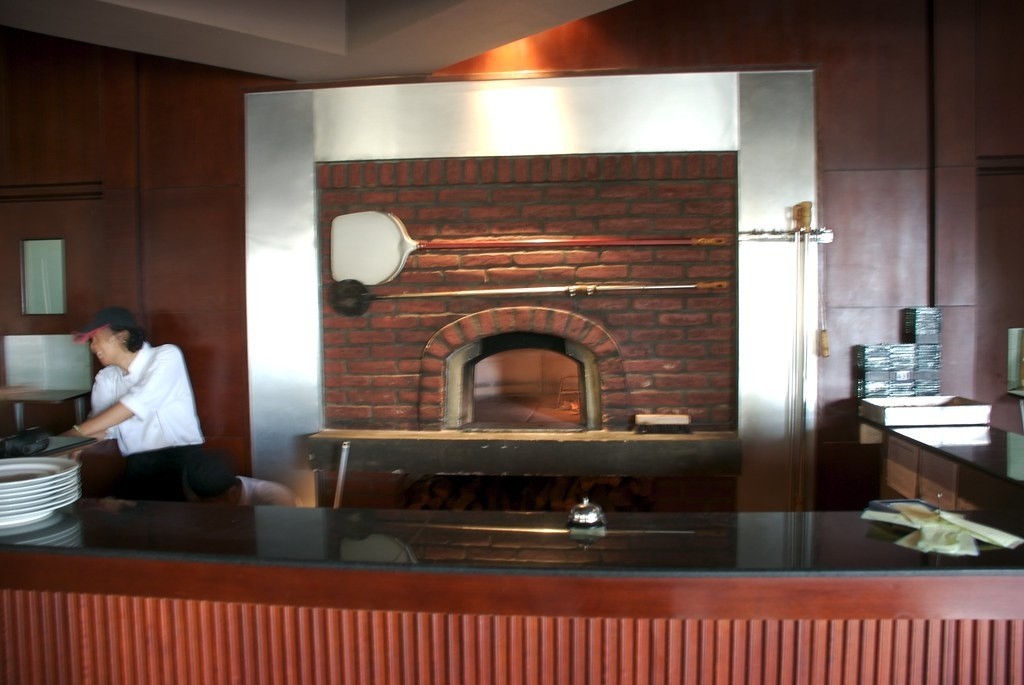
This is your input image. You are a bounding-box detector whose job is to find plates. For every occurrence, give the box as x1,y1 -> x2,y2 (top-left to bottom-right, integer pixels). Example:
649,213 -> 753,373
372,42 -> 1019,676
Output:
0,456 -> 83,528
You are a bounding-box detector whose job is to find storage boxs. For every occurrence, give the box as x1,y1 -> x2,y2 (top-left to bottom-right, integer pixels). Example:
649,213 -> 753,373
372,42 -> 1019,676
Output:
854,307 -> 992,428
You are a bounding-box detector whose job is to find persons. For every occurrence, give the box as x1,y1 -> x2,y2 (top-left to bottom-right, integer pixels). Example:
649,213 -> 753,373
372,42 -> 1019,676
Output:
182,447 -> 302,508
57,307 -> 205,500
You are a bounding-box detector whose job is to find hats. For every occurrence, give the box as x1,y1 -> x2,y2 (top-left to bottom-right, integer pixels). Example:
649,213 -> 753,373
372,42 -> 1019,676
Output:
73,306 -> 140,346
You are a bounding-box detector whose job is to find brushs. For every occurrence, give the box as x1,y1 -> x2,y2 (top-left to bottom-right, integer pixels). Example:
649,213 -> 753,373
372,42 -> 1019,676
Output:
634,414 -> 692,434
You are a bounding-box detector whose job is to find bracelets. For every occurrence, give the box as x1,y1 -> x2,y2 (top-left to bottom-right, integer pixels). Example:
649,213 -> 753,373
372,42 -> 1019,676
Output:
74,425 -> 84,438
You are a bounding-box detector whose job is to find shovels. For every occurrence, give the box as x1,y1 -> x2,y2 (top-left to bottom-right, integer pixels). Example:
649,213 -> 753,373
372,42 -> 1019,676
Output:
329,210 -> 728,286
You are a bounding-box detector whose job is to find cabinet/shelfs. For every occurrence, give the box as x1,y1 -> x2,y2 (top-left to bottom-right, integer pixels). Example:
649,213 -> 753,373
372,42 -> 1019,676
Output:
859,415 -> 1023,513
